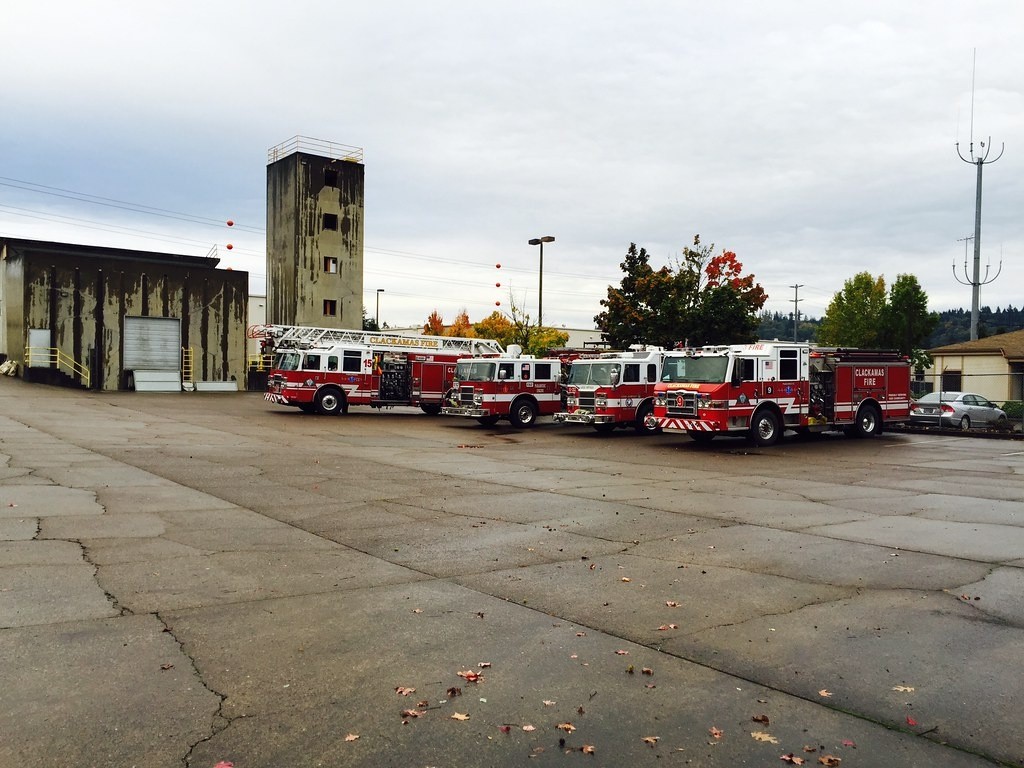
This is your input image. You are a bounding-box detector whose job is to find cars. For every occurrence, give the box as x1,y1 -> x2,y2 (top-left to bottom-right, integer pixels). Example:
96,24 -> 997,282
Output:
909,391 -> 1008,430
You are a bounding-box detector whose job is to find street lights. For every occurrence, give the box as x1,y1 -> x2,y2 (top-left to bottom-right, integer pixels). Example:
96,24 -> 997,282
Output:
528,236 -> 556,325
376,288 -> 385,328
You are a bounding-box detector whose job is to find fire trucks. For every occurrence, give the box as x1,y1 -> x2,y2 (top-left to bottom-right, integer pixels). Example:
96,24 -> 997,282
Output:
553,344 -> 666,436
652,338 -> 913,447
436,344 -> 576,428
246,322 -> 506,416
